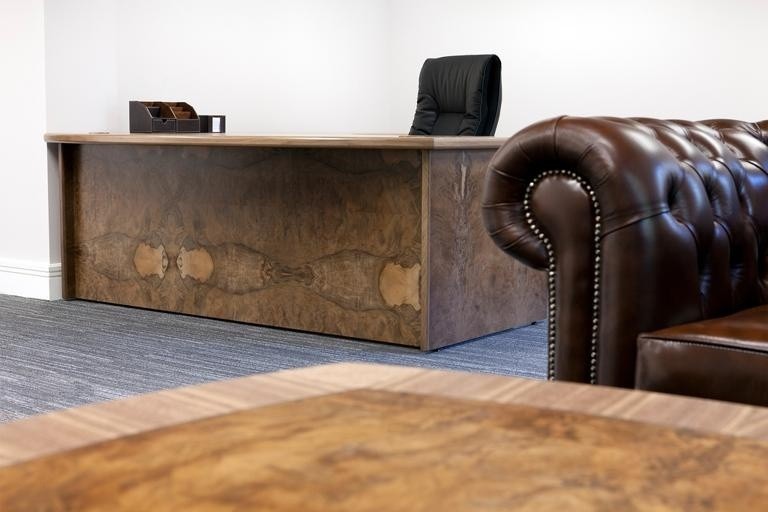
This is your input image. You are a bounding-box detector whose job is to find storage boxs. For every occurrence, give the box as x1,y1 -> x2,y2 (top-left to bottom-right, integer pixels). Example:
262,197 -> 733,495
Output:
129,101 -> 200,133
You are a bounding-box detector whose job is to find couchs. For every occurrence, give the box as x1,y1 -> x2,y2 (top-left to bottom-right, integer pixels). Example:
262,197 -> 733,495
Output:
480,115 -> 768,406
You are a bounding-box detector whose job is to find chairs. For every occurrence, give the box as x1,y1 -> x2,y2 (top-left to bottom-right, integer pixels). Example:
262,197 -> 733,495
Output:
409,54 -> 501,135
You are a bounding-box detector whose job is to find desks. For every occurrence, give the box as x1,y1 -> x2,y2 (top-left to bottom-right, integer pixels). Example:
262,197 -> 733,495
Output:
0,358 -> 768,512
